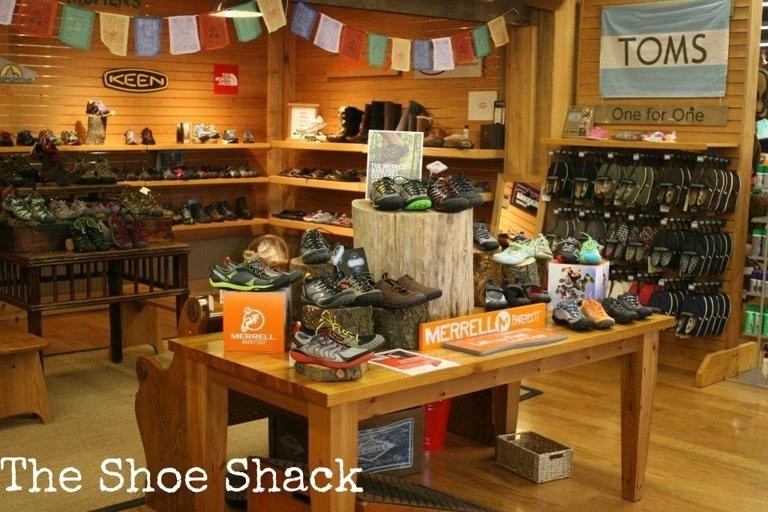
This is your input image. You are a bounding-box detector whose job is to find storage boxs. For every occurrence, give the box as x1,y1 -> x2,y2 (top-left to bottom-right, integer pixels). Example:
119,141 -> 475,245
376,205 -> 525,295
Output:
268,407 -> 425,480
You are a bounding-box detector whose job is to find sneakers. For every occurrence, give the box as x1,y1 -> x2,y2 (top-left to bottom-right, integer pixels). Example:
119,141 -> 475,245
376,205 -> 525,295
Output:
1,126 -> 264,252
542,125 -> 740,336
85,98 -> 114,120
208,221 -> 551,374
272,101 -> 495,226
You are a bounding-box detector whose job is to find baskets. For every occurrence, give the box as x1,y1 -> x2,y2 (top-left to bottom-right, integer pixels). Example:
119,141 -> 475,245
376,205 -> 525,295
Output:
493,428 -> 576,485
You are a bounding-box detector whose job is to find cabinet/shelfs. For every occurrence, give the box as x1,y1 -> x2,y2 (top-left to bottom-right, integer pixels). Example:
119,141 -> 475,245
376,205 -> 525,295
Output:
1,143 -> 270,242
269,139 -> 504,255
537,138 -> 761,388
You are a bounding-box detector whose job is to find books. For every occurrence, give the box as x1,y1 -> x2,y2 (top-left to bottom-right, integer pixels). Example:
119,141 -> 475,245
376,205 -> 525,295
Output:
443,327 -> 568,356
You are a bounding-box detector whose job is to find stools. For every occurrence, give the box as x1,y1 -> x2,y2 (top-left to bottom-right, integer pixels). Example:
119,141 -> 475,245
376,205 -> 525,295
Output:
0,324 -> 49,423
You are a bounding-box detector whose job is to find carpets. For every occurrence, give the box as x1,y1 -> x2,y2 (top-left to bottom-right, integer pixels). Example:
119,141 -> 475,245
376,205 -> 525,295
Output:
89,470 -> 499,512
0,336 -> 544,512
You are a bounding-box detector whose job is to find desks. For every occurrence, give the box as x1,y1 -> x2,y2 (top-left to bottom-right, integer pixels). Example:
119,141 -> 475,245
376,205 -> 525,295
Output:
0,240 -> 191,377
0,300 -> 29,333
134,293 -> 678,512
